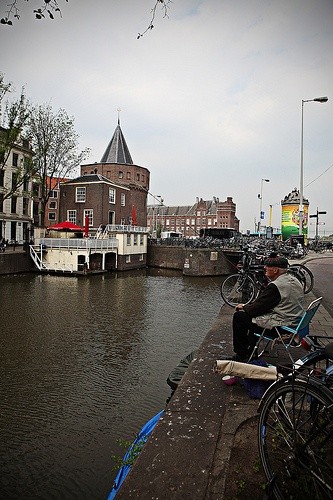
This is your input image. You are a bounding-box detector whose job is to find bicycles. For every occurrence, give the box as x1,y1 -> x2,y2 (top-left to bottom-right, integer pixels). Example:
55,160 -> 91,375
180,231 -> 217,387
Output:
158,230 -> 333,310
257,335 -> 333,500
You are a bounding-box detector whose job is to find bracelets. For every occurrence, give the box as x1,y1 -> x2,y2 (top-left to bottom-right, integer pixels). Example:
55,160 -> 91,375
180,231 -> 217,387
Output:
242,304 -> 245,307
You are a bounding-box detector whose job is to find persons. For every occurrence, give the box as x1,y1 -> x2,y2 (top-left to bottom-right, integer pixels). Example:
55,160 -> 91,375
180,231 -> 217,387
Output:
225,252 -> 304,363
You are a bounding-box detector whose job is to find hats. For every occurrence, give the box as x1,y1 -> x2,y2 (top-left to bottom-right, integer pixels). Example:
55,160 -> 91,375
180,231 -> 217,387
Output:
264,252 -> 288,268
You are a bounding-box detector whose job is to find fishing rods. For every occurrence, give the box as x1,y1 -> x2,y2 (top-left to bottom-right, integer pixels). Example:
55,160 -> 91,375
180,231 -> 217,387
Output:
127,174 -> 263,293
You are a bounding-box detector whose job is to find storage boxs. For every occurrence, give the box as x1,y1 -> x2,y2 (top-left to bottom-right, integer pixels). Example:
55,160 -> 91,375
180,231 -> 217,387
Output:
243,360 -> 273,399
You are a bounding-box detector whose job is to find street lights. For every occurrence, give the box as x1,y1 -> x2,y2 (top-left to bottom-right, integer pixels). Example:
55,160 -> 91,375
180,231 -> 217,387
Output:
298,96 -> 328,234
257,178 -> 271,238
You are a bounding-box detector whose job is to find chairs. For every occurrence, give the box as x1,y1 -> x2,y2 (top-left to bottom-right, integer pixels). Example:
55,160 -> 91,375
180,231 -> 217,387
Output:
247,297 -> 323,366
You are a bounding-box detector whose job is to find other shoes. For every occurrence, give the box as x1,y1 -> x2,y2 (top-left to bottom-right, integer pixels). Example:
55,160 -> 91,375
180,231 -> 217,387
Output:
226,354 -> 260,363
